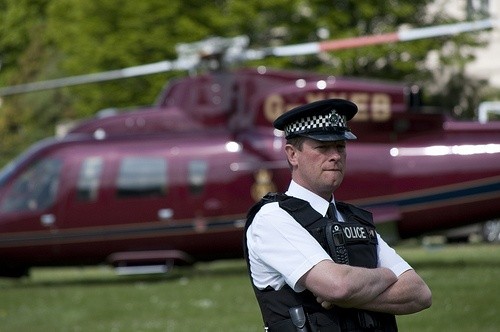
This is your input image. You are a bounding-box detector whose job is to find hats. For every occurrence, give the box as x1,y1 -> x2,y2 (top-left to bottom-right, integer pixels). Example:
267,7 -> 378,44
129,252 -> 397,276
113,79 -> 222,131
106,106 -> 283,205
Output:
274,98 -> 359,141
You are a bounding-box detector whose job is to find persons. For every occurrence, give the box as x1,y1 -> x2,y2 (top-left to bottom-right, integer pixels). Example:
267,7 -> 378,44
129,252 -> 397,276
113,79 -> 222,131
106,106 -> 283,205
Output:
243,97 -> 436,332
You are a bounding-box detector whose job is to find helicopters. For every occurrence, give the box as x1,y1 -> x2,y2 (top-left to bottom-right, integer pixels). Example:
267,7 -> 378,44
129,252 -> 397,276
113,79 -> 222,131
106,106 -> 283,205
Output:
0,19 -> 500,283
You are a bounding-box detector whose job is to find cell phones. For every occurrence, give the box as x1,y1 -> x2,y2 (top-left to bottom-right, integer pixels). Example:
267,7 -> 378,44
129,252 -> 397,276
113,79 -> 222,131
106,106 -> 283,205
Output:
326,221 -> 351,266
